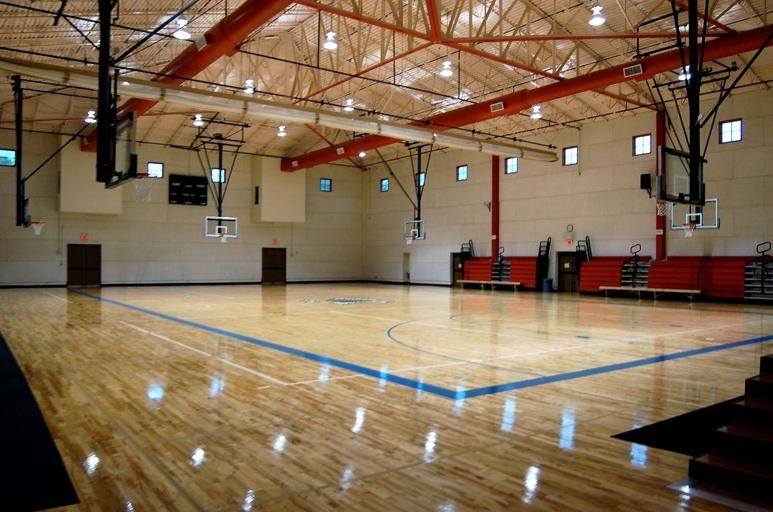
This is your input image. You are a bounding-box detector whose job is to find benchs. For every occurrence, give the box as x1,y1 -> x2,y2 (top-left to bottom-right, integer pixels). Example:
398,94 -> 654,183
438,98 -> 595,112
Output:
456,279 -> 521,291
599,286 -> 702,302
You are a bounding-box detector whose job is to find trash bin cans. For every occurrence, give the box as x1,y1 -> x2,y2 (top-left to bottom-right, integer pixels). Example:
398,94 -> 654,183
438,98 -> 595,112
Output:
543,279 -> 552,292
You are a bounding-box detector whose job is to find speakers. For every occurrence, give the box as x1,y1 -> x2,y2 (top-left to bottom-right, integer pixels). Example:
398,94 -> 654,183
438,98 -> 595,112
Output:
641,174 -> 651,189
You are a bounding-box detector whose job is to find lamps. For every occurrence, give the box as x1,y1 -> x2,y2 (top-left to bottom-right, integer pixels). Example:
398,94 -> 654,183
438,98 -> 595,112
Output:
344,96 -> 354,112
588,2 -> 606,27
172,15 -> 192,41
530,103 -> 542,119
276,123 -> 287,136
85,107 -> 98,124
192,113 -> 205,127
440,58 -> 453,78
121,80 -> 130,86
322,28 -> 339,50
243,74 -> 256,94
678,65 -> 690,80
